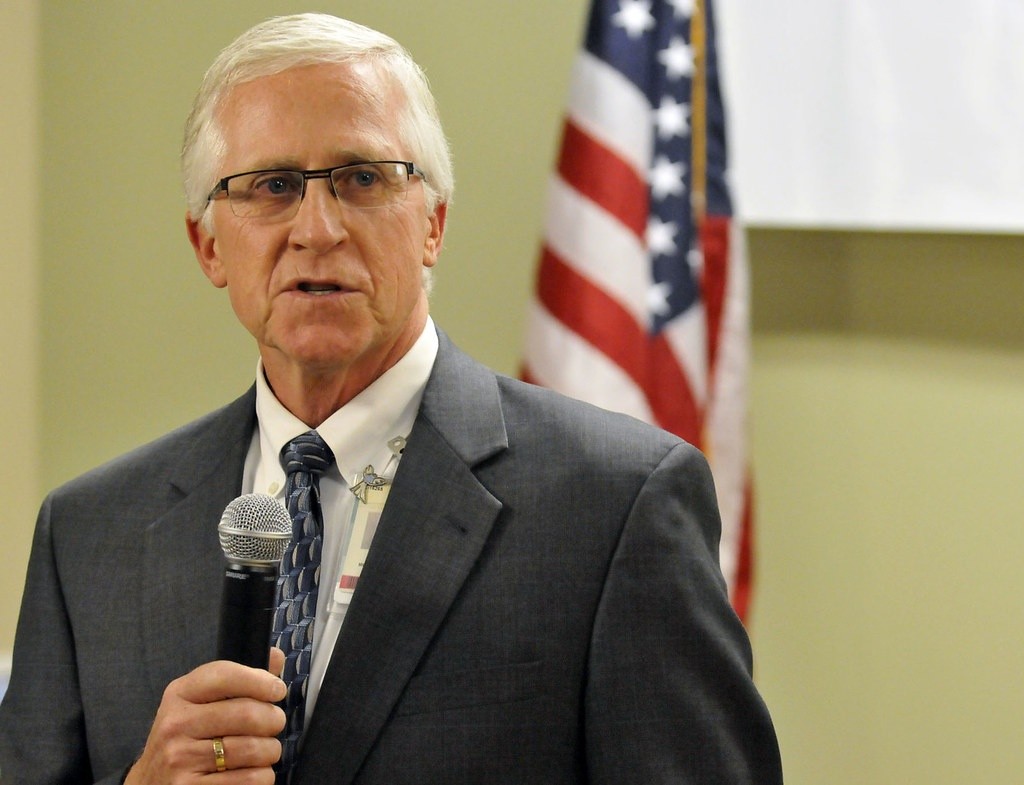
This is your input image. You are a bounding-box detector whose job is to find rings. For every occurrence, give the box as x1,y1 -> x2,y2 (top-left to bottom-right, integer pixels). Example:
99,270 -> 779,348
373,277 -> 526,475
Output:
212,735 -> 225,771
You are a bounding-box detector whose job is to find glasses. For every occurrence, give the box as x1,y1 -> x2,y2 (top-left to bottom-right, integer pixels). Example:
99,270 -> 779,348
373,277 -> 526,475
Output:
203,160 -> 427,223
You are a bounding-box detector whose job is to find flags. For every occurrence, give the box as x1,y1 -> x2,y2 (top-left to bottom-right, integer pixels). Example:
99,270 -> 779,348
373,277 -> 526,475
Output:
514,2 -> 764,629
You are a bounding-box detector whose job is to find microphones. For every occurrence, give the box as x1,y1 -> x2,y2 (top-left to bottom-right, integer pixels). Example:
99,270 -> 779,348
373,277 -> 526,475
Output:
217,492 -> 294,672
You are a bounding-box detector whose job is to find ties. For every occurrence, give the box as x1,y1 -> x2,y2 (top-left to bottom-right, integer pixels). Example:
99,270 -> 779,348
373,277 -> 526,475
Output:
271,431 -> 334,784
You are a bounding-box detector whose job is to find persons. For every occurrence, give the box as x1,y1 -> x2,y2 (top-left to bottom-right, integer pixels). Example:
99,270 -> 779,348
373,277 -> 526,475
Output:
0,11 -> 786,785
